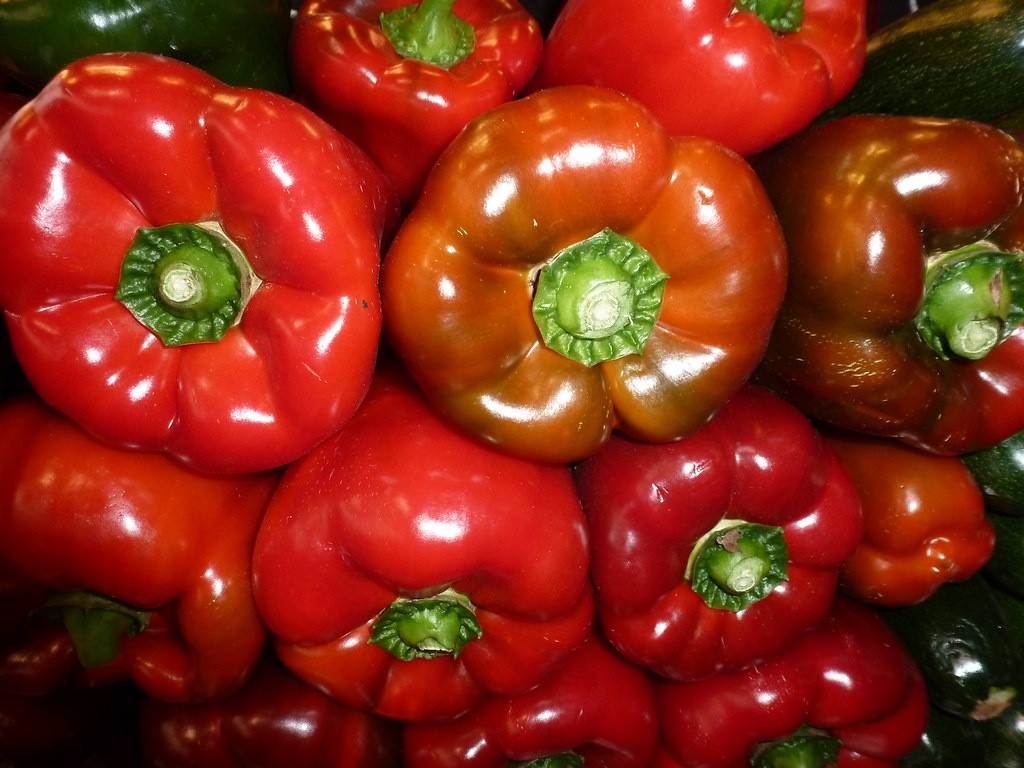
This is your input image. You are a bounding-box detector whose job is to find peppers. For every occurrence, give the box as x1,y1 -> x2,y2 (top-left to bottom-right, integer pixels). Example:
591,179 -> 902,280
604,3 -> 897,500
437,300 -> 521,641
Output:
0,0 -> 1023,768
